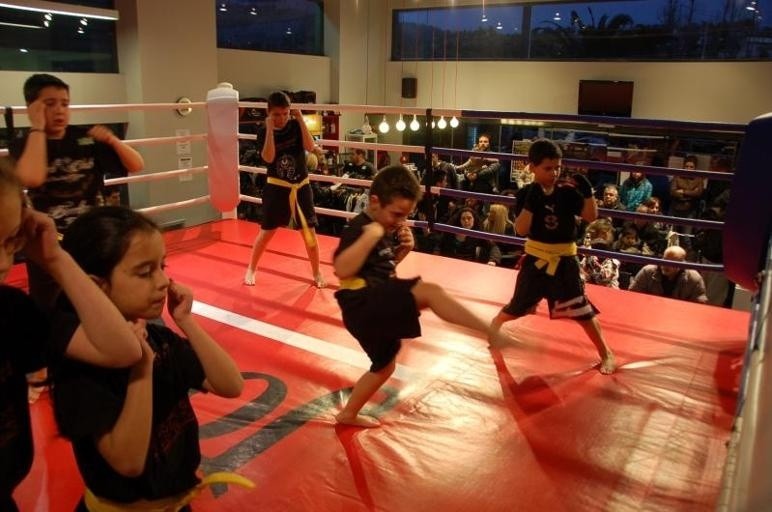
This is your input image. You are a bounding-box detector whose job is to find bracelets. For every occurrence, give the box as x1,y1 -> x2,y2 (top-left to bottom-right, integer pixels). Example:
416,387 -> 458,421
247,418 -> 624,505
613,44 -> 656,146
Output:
29,127 -> 45,132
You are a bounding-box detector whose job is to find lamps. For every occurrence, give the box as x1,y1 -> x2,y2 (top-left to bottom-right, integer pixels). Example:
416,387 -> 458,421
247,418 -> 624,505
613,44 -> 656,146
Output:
43,13 -> 90,35
361,0 -> 460,135
0,0 -> 120,21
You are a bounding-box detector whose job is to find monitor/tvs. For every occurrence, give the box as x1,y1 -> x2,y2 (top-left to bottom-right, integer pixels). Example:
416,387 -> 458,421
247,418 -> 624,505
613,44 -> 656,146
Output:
578,80 -> 634,117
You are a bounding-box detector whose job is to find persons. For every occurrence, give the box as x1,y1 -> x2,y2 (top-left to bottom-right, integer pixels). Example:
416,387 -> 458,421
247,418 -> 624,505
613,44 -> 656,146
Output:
8,74 -> 144,405
487,138 -> 616,376
47,206 -> 243,512
332,164 -> 525,428
0,156 -> 144,512
102,185 -> 122,206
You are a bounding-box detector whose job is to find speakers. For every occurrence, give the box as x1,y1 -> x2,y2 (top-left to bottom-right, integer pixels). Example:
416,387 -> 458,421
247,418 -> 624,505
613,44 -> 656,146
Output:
402,78 -> 416,98
